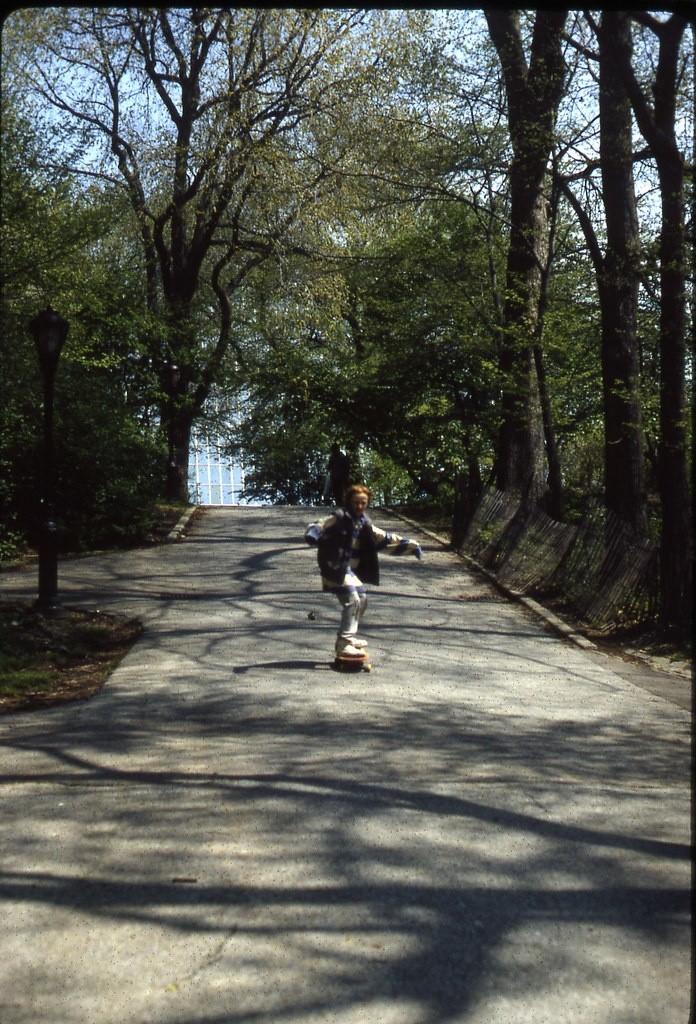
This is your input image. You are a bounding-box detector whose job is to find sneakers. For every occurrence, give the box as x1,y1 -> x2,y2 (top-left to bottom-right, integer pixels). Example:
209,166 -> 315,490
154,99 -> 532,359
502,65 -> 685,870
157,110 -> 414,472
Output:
335,635 -> 368,648
337,640 -> 365,657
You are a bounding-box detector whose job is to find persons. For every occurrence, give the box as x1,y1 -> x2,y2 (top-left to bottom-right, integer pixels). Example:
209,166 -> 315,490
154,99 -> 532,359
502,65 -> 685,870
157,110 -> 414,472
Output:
325,443 -> 349,507
304,484 -> 422,659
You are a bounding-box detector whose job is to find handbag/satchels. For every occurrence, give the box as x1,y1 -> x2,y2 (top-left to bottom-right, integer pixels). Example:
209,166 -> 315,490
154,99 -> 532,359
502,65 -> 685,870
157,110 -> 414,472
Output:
323,476 -> 331,495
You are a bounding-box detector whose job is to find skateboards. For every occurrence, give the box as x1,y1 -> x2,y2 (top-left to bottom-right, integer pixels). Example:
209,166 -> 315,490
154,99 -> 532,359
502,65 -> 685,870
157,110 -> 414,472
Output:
336,647 -> 372,673
